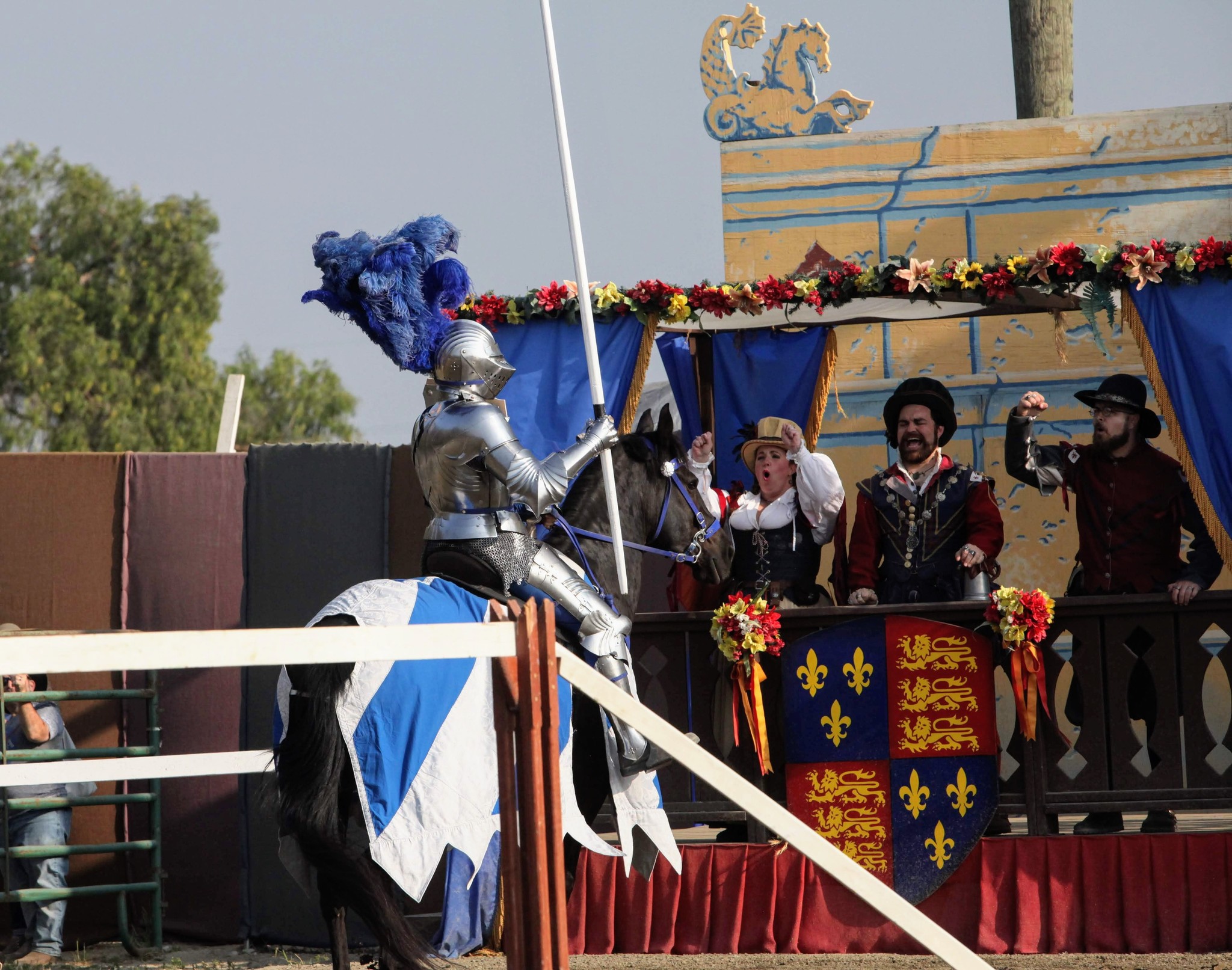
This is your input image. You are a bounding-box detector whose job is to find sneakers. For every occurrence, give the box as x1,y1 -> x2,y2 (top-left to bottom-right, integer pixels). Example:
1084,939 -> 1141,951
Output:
5,945 -> 62,967
1073,812 -> 1124,833
1140,810 -> 1177,833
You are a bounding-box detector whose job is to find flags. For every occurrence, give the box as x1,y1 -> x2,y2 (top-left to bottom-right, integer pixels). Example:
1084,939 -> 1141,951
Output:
1120,275 -> 1232,571
487,311 -> 659,541
655,321 -> 839,491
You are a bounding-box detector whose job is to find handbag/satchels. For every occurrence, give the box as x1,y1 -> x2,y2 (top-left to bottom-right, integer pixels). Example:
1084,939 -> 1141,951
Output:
63,726 -> 98,797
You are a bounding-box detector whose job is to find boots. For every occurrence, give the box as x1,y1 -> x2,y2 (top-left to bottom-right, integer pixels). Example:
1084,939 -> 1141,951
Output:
594,654 -> 701,776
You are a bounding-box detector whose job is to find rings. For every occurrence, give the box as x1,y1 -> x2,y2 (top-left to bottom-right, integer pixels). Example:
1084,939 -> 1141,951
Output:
1173,587 -> 1180,590
856,594 -> 861,599
794,427 -> 795,429
1187,587 -> 1192,589
962,544 -> 975,556
1026,394 -> 1036,400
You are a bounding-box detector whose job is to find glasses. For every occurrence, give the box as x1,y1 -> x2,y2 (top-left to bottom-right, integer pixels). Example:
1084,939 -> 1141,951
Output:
1088,406 -> 1135,417
3,677 -> 11,686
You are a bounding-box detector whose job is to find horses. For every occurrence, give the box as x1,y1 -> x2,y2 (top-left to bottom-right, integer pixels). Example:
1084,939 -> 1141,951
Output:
263,404 -> 734,970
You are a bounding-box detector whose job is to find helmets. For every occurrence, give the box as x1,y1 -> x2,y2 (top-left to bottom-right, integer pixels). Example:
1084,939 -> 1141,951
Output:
432,319 -> 516,401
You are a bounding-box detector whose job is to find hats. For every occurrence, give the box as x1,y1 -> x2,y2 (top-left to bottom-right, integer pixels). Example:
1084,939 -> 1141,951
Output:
883,377 -> 957,449
732,417 -> 805,477
1074,374 -> 1161,439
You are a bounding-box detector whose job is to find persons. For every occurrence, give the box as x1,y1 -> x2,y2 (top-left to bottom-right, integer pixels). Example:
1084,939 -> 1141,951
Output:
685,417 -> 844,843
1004,373 -> 1224,836
0,674 -> 72,967
848,377 -> 1013,836
412,319 -> 701,778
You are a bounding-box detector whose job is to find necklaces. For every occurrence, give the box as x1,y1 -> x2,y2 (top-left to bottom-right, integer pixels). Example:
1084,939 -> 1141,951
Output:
904,464 -> 935,480
761,496 -> 773,507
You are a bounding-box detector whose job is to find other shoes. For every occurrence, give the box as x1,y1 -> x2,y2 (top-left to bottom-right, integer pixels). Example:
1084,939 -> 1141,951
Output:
717,821 -> 747,842
982,812 -> 1011,837
